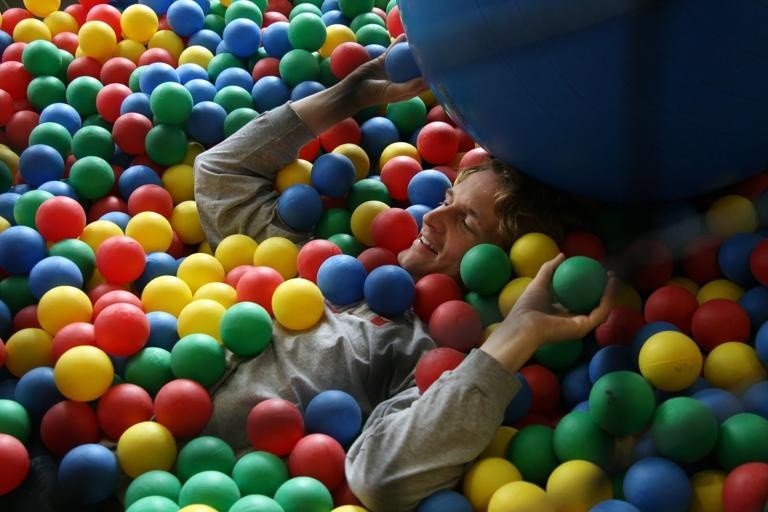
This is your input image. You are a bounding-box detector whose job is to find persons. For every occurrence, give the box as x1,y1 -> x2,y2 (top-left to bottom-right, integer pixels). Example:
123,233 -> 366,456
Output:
192,34 -> 618,512
